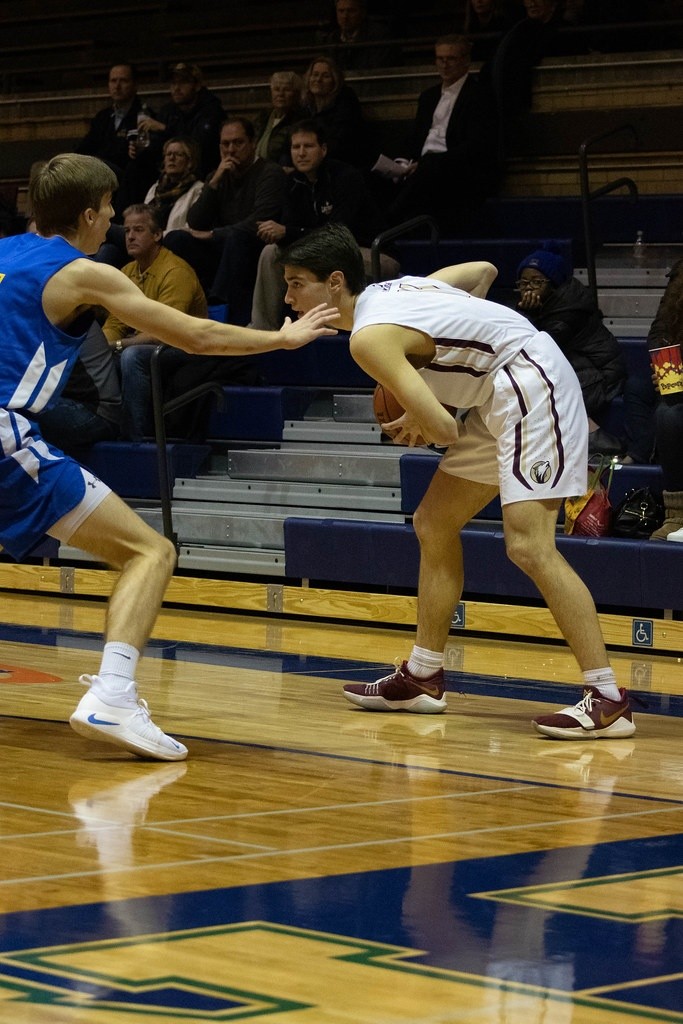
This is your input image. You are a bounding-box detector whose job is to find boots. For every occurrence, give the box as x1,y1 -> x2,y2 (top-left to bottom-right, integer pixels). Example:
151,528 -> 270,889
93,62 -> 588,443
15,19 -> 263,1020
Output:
651,488 -> 683,540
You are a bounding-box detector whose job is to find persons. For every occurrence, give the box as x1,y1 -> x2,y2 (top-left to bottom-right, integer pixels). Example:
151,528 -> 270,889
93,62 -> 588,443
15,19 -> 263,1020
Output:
512,249 -> 683,542
28,0 -> 576,442
276,226 -> 636,738
0,153 -> 342,760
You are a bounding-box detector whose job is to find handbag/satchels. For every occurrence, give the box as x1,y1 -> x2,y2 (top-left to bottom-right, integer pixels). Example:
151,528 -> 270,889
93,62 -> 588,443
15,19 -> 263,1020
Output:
612,485 -> 662,539
562,453 -> 615,539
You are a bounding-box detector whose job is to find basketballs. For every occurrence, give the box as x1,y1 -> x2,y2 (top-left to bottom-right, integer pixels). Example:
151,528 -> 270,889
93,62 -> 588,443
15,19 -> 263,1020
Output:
373,382 -> 458,447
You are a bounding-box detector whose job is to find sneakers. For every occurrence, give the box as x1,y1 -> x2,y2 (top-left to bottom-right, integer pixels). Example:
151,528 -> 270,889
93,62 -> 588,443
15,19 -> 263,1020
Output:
342,702 -> 445,752
67,761 -> 191,830
343,662 -> 446,714
531,694 -> 637,742
531,737 -> 636,779
67,674 -> 188,762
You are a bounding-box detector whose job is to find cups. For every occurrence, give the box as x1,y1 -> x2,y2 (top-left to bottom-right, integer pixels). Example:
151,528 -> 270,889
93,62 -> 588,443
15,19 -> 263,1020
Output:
648,345 -> 683,396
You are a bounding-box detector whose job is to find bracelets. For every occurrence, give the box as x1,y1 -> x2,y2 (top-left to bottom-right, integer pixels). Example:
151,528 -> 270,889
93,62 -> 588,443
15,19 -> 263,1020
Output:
116,339 -> 123,351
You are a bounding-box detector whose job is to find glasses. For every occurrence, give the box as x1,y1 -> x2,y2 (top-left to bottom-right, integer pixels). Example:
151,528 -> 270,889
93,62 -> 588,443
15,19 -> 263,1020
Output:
513,279 -> 548,291
162,151 -> 186,161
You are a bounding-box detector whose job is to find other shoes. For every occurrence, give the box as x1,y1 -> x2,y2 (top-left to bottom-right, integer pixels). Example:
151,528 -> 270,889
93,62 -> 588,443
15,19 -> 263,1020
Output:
666,526 -> 683,543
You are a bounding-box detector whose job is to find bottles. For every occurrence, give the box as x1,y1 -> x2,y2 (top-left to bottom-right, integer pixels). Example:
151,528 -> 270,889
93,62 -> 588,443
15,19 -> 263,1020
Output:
634,230 -> 643,258
135,103 -> 150,146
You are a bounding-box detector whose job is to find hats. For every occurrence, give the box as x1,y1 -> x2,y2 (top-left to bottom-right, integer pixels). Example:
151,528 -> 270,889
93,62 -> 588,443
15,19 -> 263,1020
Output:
519,251 -> 572,288
164,63 -> 201,81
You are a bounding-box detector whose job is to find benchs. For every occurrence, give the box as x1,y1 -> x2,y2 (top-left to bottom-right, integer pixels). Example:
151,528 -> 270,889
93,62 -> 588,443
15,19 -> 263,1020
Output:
0,1 -> 683,617
